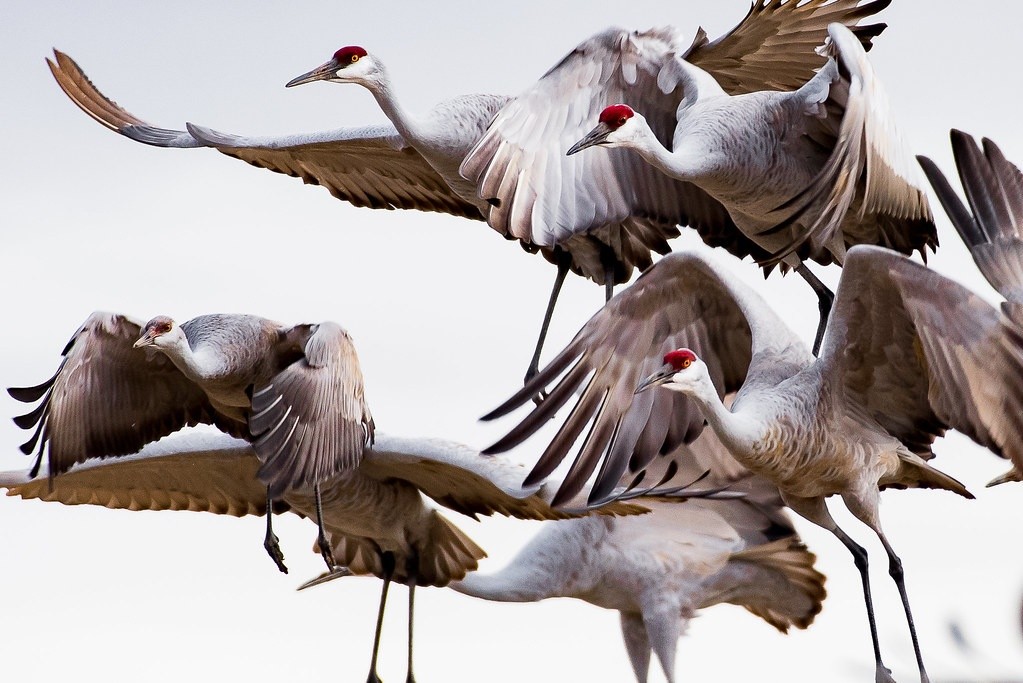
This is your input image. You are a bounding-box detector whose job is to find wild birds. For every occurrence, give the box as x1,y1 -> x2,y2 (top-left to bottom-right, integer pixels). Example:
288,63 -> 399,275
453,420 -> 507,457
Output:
2,428 -> 748,683
292,495 -> 829,683
458,22 -> 939,357
8,309 -> 374,574
477,244 -> 1023,683
916,128 -> 1023,488
44,0 -> 891,420
